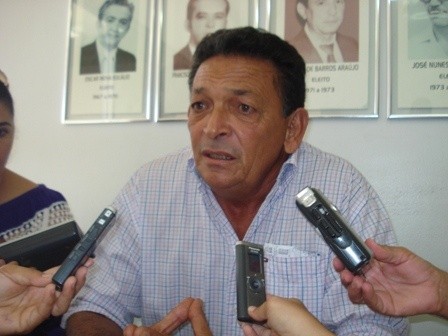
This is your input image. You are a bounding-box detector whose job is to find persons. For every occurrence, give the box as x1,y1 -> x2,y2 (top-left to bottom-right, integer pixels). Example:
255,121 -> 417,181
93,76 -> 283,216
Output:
242,293 -> 336,336
61,26 -> 410,336
286,0 -> 358,63
333,238 -> 447,319
1,258 -> 94,335
409,0 -> 448,60
0,71 -> 82,336
175,0 -> 230,70
81,0 -> 135,74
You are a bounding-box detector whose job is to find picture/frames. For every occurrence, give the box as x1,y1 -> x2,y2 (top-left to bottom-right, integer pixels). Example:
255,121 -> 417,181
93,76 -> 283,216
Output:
387,0 -> 448,118
266,0 -> 379,119
154,0 -> 259,122
60,0 -> 155,124
